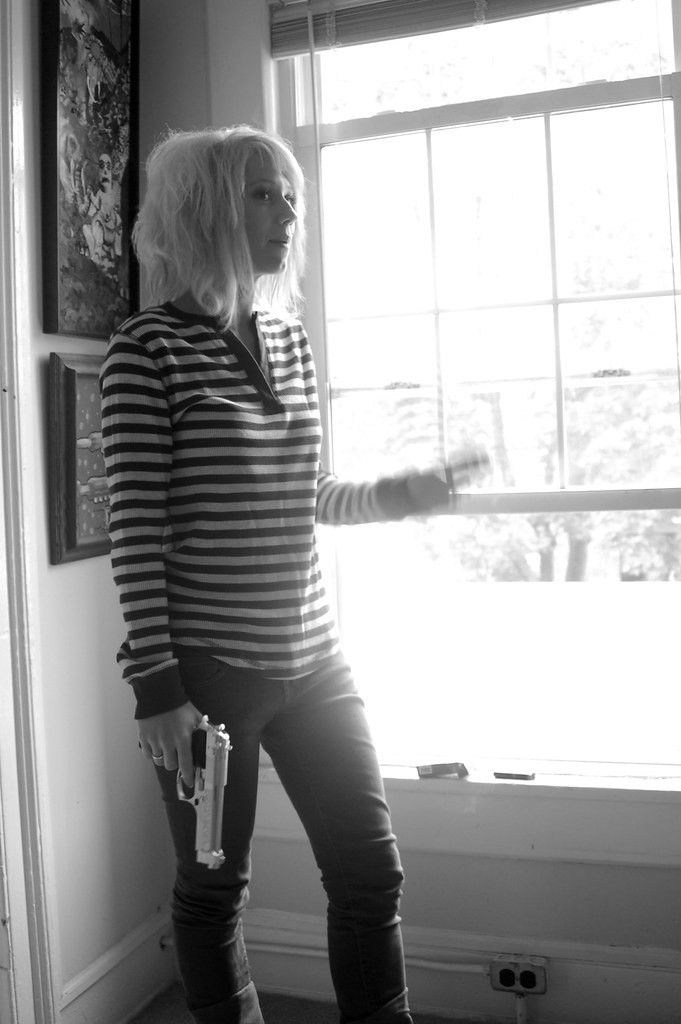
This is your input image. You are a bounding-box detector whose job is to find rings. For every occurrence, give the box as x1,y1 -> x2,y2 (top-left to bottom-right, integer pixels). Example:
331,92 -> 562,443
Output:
152,754 -> 163,760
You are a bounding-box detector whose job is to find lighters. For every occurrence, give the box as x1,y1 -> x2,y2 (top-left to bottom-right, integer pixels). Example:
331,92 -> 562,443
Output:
494,771 -> 535,780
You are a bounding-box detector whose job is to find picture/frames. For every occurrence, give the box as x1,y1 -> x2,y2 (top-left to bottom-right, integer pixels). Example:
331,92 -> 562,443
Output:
39,1 -> 140,338
48,351 -> 117,567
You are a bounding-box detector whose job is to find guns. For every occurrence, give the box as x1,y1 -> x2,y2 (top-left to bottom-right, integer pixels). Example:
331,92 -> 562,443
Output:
138,714 -> 234,871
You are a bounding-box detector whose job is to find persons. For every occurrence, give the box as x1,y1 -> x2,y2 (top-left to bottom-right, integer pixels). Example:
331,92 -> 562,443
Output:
98,125 -> 495,1024
